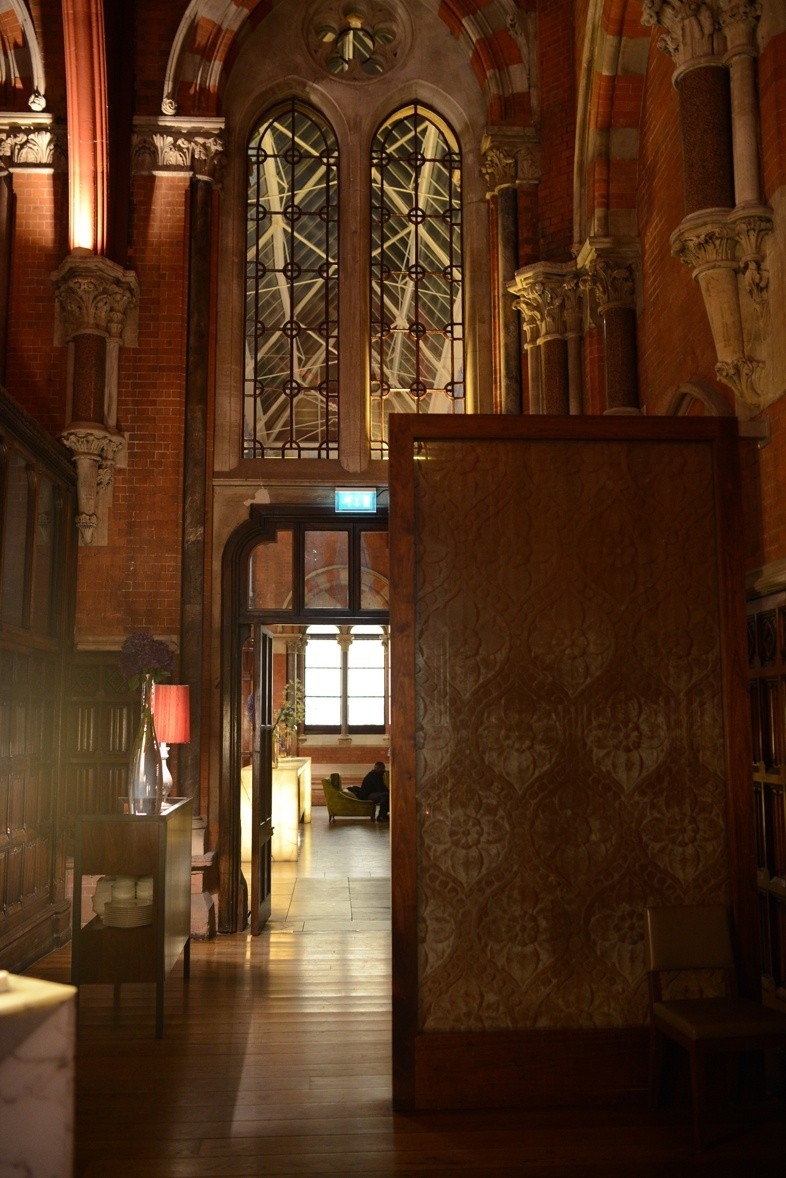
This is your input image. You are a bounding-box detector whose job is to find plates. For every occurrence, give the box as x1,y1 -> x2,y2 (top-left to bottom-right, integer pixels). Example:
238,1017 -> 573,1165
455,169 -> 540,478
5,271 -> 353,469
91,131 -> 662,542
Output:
105,901 -> 153,928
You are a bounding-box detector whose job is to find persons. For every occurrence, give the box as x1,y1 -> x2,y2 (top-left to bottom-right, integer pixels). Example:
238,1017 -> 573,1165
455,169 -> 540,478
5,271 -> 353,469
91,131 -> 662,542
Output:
360,761 -> 389,822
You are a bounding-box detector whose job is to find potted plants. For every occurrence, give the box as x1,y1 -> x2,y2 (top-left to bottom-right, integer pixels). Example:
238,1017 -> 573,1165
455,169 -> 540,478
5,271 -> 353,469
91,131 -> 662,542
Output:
269,678 -> 305,768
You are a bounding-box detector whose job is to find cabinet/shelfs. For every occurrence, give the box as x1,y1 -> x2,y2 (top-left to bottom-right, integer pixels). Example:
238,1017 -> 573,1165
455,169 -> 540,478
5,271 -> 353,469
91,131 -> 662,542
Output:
73,797 -> 192,1041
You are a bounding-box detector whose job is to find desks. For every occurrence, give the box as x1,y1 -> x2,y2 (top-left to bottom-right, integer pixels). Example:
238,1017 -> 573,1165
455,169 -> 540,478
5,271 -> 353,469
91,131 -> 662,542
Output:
0,969 -> 78,1178
242,757 -> 315,859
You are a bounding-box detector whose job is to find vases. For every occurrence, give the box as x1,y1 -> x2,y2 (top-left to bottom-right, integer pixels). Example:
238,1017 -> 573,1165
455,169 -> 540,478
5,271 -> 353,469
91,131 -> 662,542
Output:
128,673 -> 164,817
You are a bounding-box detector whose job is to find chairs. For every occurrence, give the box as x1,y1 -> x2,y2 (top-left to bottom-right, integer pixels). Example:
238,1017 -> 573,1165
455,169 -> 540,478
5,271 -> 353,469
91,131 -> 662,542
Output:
323,773 -> 378,824
643,904 -> 785,1151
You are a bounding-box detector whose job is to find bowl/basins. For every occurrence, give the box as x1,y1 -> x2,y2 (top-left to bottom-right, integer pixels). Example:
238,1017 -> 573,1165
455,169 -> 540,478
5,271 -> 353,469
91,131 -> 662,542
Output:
92,875 -> 153,918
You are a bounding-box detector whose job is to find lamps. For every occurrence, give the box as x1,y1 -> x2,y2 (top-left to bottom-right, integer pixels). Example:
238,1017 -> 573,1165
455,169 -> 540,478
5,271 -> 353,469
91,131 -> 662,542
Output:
151,684 -> 191,809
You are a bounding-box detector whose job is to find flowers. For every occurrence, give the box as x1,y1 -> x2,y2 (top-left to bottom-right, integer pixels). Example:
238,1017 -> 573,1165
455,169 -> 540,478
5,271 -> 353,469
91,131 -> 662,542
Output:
115,628 -> 174,687
